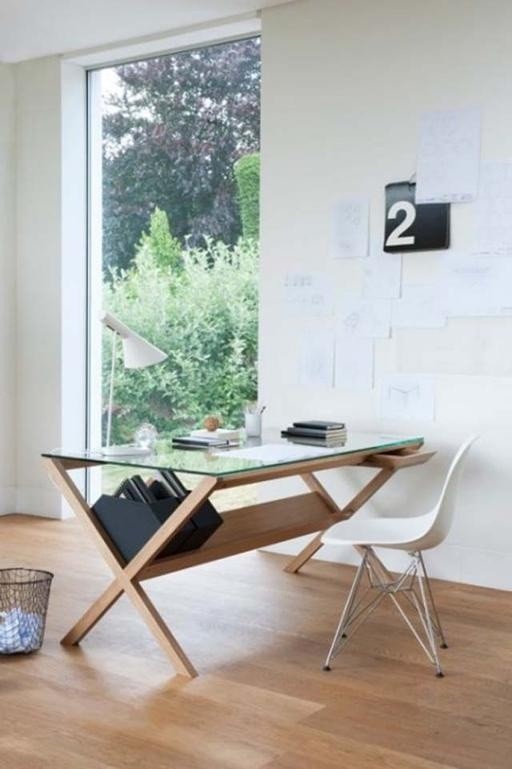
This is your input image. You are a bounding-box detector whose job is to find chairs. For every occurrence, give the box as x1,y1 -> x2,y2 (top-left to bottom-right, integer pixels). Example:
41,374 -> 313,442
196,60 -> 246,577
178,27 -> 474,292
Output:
320,431 -> 481,677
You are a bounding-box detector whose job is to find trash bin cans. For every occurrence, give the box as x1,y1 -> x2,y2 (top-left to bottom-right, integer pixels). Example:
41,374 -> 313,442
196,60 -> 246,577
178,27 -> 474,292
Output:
0,568 -> 54,656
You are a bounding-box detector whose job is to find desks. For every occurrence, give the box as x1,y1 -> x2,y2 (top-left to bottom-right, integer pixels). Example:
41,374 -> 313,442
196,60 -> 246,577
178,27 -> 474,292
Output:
40,427 -> 438,680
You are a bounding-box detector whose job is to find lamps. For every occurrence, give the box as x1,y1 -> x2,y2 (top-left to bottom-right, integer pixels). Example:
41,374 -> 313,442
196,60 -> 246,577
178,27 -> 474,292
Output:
99,309 -> 169,456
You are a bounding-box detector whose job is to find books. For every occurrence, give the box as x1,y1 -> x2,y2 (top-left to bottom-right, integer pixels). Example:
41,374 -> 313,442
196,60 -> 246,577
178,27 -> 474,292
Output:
170,426 -> 245,449
289,439 -> 346,448
281,420 -> 347,438
113,468 -> 188,504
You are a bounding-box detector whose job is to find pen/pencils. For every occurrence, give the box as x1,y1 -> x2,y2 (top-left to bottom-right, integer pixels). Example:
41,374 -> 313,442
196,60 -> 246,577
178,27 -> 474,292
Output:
245,403 -> 266,414
